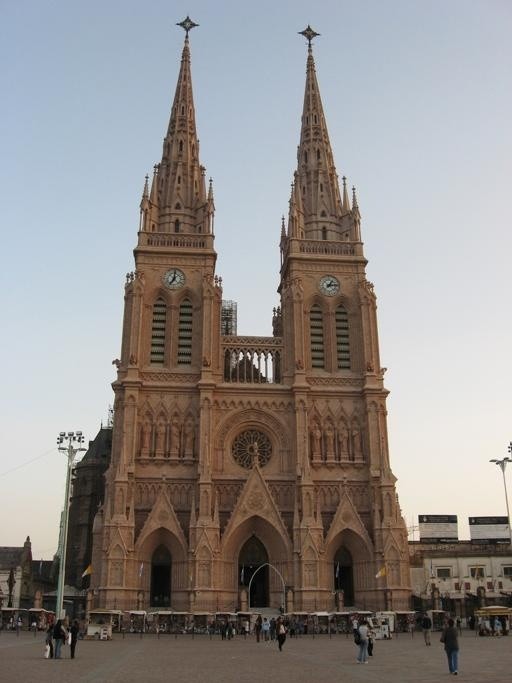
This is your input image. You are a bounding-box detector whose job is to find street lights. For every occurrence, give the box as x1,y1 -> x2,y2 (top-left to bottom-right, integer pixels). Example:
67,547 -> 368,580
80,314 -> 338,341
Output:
486,456 -> 512,544
507,439 -> 512,455
53,428 -> 89,627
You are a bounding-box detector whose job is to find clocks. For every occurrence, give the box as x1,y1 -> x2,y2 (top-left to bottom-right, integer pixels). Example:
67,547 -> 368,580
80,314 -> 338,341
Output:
318,273 -> 341,298
162,266 -> 187,290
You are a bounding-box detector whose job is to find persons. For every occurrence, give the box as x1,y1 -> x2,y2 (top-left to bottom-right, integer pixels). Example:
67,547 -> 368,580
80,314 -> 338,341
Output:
210,618 -> 305,652
422,613 -> 432,646
43,620 -> 79,659
351,615 -> 375,664
441,618 -> 461,675
477,616 -> 511,637
